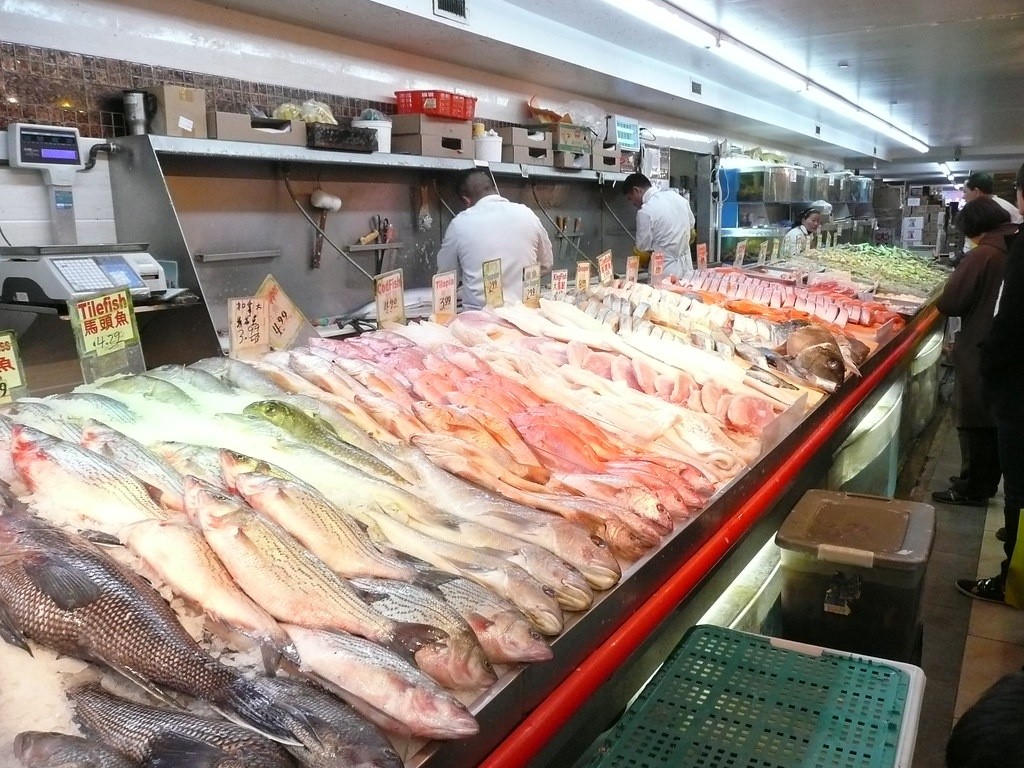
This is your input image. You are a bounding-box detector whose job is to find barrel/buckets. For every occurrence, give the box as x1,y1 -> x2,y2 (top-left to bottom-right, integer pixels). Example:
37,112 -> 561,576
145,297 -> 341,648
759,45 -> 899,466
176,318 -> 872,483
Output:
474,135 -> 502,162
350,120 -> 392,153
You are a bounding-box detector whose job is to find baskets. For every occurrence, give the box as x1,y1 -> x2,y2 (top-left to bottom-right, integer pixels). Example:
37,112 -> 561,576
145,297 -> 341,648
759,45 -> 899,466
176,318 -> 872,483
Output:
571,625 -> 934,768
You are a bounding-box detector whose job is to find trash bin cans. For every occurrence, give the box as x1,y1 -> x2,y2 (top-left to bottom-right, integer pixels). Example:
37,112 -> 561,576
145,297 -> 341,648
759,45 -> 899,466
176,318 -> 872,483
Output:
774,489 -> 938,660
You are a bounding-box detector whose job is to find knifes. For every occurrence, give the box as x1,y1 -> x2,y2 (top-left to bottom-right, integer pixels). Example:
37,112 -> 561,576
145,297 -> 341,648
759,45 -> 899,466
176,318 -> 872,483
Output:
559,216 -> 570,262
557,214 -> 563,251
336,229 -> 380,262
380,223 -> 399,277
569,216 -> 583,263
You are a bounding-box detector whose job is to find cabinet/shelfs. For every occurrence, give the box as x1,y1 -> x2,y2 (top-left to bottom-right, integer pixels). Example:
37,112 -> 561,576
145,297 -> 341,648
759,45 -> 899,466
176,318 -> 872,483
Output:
1,130 -> 952,767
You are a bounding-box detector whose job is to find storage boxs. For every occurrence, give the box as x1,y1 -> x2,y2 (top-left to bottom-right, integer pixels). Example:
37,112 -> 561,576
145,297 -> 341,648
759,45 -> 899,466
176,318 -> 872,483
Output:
392,134 -> 477,159
776,488 -> 939,638
554,152 -> 590,169
523,124 -> 592,154
590,140 -> 622,157
502,145 -> 555,166
389,114 -> 474,139
306,122 -> 379,152
494,127 -> 553,150
141,85 -> 208,139
206,111 -> 308,147
394,90 -> 479,121
590,155 -> 621,171
573,623 -> 927,768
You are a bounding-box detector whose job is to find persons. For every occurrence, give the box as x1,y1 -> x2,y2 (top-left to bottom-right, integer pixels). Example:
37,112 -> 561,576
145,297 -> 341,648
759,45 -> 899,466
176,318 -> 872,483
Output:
779,208 -> 821,259
436,170 -> 554,314
953,162 -> 1023,603
622,173 -> 695,284
961,171 -> 1024,254
934,196 -> 1020,507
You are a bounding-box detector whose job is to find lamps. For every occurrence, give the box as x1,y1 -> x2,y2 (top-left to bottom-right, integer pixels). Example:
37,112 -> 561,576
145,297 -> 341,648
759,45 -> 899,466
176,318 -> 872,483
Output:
938,163 -> 960,191
605,0 -> 928,156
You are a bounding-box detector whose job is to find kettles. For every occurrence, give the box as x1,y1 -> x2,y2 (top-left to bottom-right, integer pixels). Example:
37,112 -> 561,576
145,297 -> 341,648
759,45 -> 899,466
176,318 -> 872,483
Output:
122,89 -> 157,136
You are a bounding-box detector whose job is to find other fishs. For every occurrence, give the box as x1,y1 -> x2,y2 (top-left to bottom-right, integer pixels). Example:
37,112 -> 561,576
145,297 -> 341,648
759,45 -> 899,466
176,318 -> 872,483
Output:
0,268 -> 908,768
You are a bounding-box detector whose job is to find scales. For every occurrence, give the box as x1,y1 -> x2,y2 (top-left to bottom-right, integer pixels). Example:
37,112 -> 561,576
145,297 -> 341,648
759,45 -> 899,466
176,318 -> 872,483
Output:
0,121 -> 169,317
